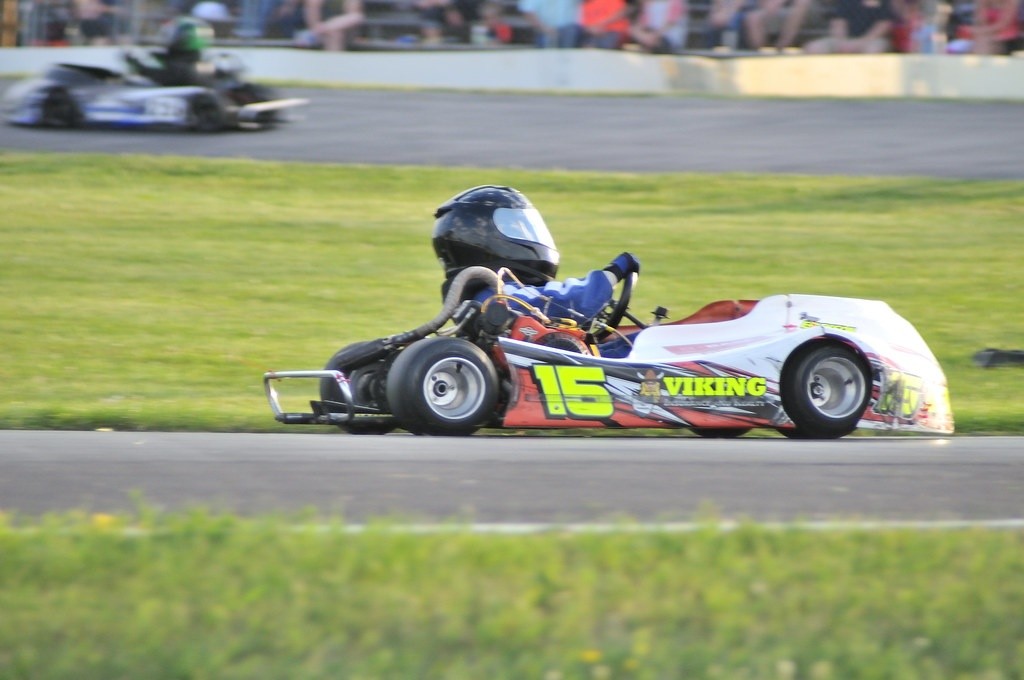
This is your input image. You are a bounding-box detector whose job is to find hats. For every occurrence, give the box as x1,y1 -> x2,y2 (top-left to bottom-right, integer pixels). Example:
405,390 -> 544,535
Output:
191,0 -> 233,23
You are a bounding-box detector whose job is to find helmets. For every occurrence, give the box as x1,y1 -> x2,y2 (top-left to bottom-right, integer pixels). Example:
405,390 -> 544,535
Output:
433,184 -> 559,282
171,18 -> 216,53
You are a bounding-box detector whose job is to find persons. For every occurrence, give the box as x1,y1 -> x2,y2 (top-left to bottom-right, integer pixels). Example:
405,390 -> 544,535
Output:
125,17 -> 230,86
0,1 -> 1023,56
432,185 -> 642,359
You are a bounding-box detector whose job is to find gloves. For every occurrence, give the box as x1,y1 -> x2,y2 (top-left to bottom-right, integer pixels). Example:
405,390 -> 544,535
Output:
603,252 -> 640,284
